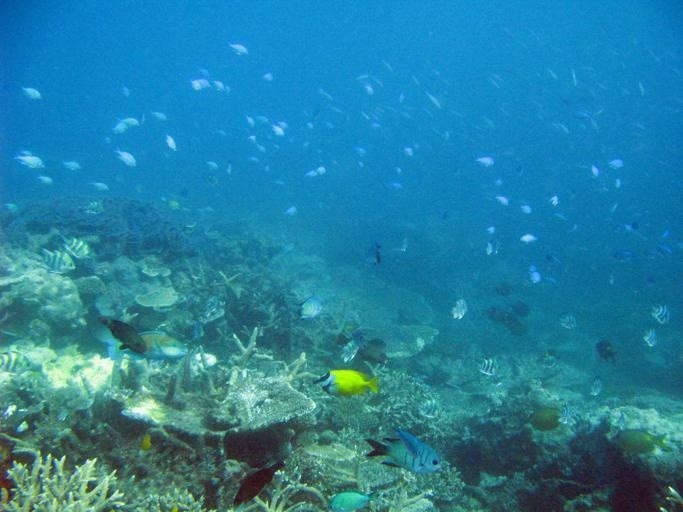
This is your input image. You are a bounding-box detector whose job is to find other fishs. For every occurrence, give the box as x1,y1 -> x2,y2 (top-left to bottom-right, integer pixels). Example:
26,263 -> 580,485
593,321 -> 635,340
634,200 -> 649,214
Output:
0,41 -> 683,399
